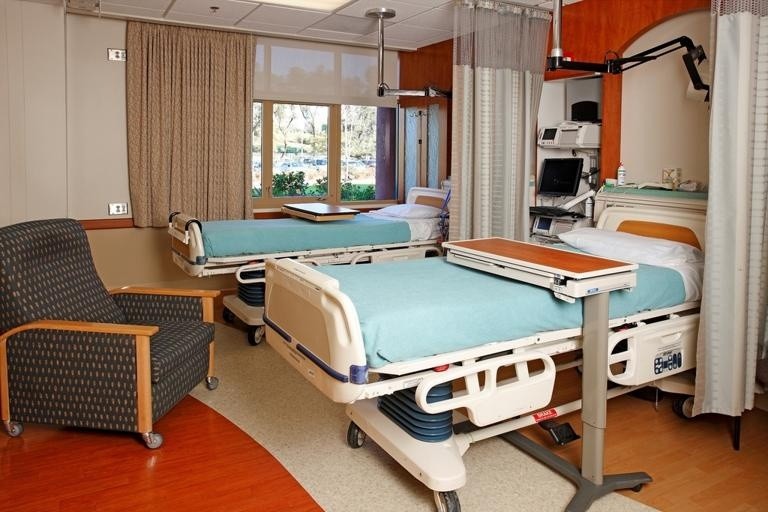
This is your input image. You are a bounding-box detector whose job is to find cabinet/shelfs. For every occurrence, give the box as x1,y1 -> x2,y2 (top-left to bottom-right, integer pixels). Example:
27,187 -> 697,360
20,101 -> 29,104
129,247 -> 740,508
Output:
395,101 -> 448,202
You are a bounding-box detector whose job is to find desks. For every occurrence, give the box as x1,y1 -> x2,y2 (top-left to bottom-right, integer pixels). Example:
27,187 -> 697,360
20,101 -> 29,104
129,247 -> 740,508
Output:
442,237 -> 658,511
280,200 -> 360,222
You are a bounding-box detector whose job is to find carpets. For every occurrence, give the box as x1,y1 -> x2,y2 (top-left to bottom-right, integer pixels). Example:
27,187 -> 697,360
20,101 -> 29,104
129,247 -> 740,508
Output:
187,321 -> 660,512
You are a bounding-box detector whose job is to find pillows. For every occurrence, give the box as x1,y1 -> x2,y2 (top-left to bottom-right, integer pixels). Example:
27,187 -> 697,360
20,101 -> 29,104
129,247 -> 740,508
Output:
379,204 -> 447,219
557,228 -> 704,267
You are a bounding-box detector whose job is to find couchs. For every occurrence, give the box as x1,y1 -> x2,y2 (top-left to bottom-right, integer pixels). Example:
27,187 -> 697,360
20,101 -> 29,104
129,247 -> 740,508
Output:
0,218 -> 221,450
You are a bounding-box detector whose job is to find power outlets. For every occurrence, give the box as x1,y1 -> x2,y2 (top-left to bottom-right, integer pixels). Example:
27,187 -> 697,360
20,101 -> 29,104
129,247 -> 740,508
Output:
108,201 -> 127,215
108,48 -> 126,61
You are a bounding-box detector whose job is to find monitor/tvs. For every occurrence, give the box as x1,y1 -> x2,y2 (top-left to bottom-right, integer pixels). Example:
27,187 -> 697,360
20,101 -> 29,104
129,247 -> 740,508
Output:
536,157 -> 585,198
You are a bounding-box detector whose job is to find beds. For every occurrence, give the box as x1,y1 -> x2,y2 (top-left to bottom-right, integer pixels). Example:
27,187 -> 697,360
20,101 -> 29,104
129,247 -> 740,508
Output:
171,187 -> 451,344
265,208 -> 767,511
